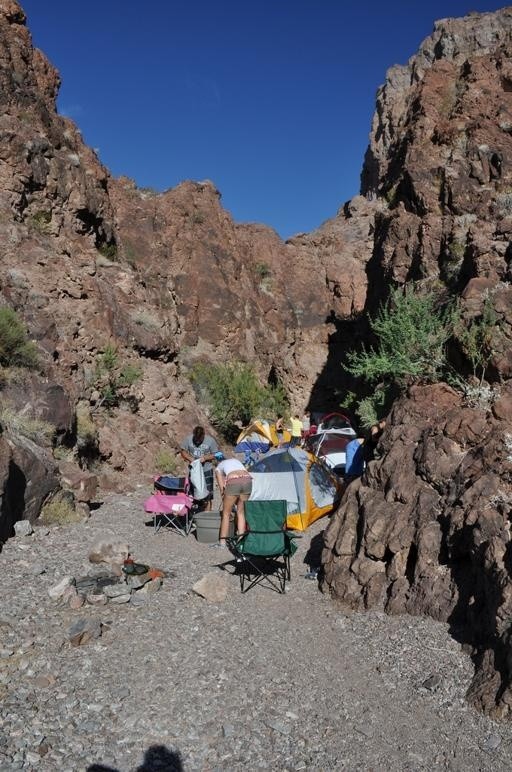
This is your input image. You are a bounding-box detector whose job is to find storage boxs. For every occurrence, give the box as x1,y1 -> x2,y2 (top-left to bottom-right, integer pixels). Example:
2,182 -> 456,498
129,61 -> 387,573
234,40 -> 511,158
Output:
193,511 -> 234,543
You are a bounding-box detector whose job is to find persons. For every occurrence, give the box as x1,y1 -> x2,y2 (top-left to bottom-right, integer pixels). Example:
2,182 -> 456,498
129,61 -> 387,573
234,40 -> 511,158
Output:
214,450 -> 253,544
302,410 -> 311,447
232,415 -> 247,447
275,414 -> 287,449
179,425 -> 220,512
361,418 -> 386,467
289,412 -> 303,447
345,437 -> 366,476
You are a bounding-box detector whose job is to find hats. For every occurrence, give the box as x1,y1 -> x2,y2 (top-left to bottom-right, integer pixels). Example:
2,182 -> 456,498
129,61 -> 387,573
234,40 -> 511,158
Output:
193,426 -> 204,446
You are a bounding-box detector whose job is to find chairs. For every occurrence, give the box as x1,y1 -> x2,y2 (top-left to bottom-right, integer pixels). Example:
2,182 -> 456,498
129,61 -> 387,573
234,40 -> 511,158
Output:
231,500 -> 297,594
143,475 -> 200,538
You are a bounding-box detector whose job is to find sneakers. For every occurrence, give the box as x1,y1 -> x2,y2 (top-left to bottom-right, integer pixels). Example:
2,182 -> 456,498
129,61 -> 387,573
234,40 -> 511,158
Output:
208,543 -> 226,549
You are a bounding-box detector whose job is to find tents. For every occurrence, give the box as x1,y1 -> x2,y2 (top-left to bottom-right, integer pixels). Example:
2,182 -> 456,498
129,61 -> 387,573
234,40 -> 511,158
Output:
301,412 -> 358,475
245,446 -> 348,533
233,416 -> 292,454
